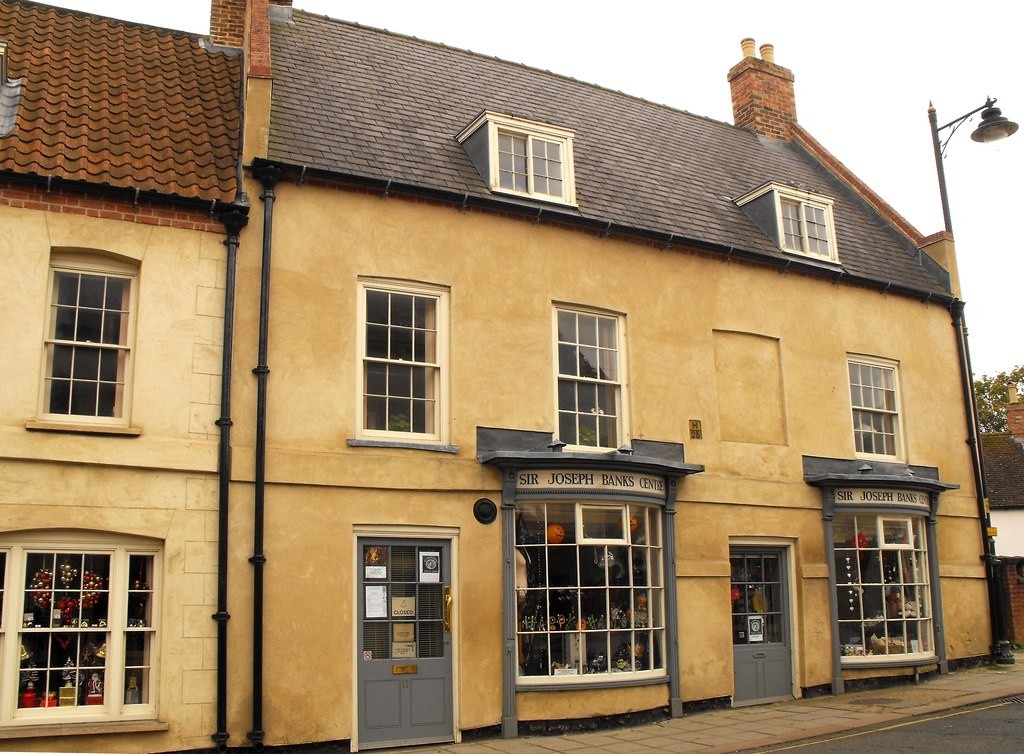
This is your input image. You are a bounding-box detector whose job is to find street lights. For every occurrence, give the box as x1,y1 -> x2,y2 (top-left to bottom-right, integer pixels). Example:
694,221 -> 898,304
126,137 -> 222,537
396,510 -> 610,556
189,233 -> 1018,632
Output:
927,95 -> 1018,664
